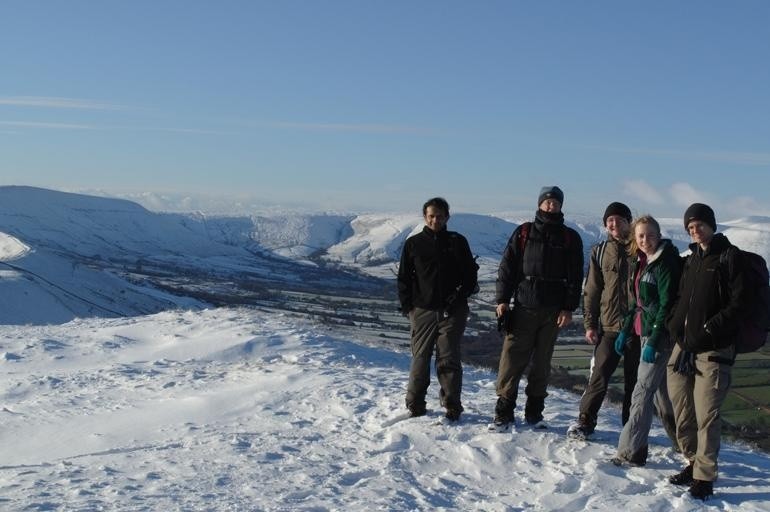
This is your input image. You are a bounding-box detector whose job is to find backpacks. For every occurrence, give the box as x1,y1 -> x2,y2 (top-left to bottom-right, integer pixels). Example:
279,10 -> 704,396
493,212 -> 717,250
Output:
716,243 -> 769,354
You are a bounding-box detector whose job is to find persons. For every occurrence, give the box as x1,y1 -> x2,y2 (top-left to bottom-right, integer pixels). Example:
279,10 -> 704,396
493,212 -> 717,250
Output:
663,202 -> 749,505
398,197 -> 479,423
605,216 -> 681,468
566,202 -> 641,439
490,185 -> 584,432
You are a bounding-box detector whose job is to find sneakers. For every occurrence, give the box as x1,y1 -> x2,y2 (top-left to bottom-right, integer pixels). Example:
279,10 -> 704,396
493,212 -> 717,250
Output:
603,455 -> 646,468
524,414 -> 548,430
444,408 -> 461,423
668,464 -> 693,484
682,479 -> 714,503
487,414 -> 515,434
566,416 -> 597,440
407,409 -> 426,419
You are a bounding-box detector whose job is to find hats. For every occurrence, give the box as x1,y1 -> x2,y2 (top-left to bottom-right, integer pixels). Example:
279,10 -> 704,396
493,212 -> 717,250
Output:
683,202 -> 716,233
602,201 -> 633,227
538,185 -> 564,208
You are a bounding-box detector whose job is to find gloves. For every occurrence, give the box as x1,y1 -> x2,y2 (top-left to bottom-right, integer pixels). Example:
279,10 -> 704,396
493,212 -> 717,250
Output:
615,331 -> 625,356
642,344 -> 655,363
493,309 -> 513,337
